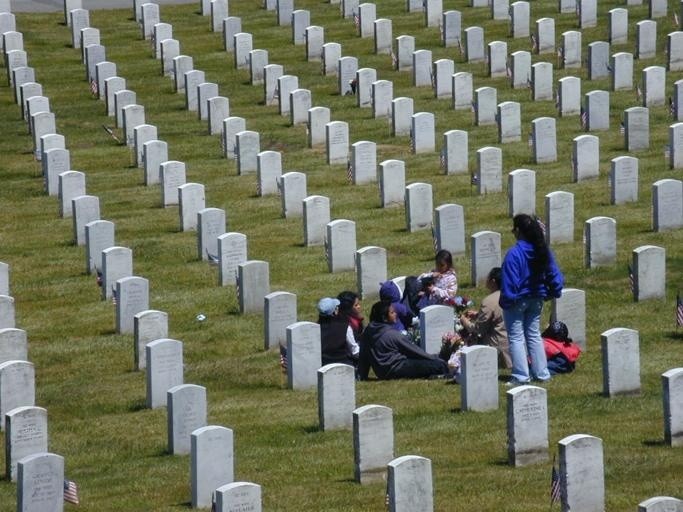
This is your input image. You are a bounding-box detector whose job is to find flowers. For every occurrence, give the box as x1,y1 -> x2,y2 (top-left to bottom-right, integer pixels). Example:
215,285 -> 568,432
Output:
400,295 -> 476,361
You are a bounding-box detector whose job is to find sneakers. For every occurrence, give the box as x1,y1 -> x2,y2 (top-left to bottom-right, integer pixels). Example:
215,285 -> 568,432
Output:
505,377 -> 529,388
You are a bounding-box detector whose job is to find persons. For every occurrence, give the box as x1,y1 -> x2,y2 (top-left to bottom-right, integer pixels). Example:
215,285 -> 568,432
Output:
497,211 -> 564,387
313,249 -> 585,379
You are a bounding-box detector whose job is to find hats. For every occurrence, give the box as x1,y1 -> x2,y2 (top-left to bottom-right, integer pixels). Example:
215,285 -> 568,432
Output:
317,297 -> 341,316
